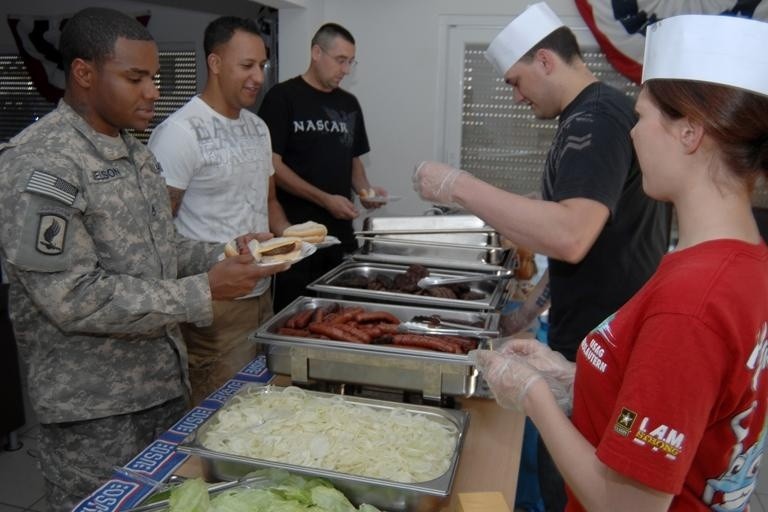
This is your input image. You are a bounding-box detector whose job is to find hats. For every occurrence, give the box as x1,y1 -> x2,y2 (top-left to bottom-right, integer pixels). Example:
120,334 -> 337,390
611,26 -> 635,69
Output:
484,2 -> 565,78
640,15 -> 767,97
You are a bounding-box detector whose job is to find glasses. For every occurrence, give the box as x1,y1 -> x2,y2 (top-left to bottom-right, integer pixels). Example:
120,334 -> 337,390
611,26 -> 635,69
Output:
321,47 -> 357,67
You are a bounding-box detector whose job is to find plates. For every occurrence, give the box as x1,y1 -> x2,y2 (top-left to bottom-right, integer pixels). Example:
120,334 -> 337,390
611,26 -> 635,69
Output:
358,192 -> 405,204
306,234 -> 340,253
216,240 -> 317,274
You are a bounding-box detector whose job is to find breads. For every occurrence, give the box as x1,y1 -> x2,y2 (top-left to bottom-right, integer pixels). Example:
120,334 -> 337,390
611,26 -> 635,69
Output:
259,240 -> 301,260
283,220 -> 327,243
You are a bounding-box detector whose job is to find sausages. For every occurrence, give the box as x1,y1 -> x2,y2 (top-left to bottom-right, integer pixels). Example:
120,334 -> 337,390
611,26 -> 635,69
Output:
279,304 -> 478,355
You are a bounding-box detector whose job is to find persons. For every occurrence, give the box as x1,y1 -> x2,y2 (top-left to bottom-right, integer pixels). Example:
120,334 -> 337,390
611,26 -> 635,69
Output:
1,6 -> 292,512
412,27 -> 675,512
146,17 -> 295,411
465,78 -> 768,511
255,24 -> 388,317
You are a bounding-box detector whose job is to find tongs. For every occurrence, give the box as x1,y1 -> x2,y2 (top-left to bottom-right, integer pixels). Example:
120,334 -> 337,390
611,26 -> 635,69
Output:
417,268 -> 514,291
394,314 -> 499,341
118,466 -> 291,512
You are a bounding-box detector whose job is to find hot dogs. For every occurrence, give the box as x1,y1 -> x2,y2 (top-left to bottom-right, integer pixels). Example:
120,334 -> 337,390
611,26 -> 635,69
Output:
223,235 -> 262,263
